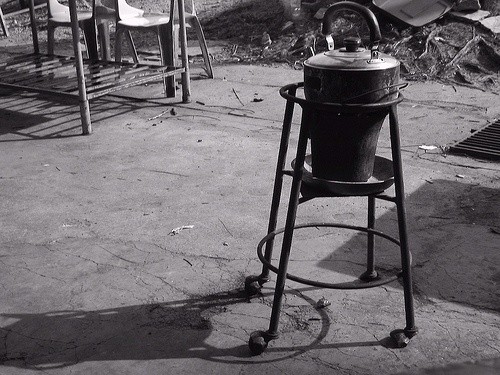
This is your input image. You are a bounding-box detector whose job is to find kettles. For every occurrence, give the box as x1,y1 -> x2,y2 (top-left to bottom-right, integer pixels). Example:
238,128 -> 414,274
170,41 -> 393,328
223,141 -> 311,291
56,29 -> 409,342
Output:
299,1 -> 401,112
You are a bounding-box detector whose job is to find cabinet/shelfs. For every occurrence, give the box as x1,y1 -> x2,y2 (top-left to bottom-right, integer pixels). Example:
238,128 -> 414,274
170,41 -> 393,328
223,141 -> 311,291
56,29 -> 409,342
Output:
243,79 -> 420,356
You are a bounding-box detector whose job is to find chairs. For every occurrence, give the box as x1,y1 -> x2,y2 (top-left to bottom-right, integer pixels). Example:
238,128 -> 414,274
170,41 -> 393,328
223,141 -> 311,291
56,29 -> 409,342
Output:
115,0 -> 215,97
47,0 -> 141,69
116,0 -> 145,67
